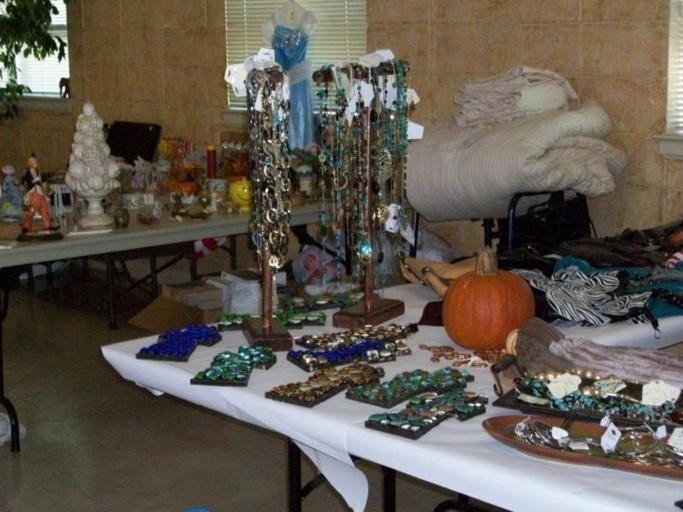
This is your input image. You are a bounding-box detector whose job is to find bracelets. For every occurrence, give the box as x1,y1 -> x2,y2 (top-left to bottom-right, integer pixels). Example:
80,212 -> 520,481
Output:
519,419 -> 572,452
615,426 -> 661,460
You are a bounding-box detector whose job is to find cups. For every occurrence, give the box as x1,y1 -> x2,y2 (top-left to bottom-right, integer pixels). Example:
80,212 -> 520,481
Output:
113,209 -> 129,226
229,181 -> 250,211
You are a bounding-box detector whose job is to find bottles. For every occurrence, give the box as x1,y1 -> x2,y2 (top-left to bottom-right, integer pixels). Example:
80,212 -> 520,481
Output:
205,144 -> 216,178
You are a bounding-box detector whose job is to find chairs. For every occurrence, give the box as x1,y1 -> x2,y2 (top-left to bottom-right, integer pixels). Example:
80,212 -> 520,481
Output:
1,348 -> 21,454
469,189 -> 592,257
106,121 -> 162,167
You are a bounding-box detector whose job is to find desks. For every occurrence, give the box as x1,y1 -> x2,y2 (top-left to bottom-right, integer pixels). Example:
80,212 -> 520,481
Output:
0,200 -> 419,454
100,282 -> 683,512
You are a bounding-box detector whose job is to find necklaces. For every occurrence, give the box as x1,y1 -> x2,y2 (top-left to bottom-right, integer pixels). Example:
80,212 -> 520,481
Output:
243,64 -> 292,315
316,55 -> 409,299
532,365 -> 620,399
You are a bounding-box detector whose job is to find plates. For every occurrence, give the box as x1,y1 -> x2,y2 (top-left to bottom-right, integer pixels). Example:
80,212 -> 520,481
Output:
481,415 -> 683,476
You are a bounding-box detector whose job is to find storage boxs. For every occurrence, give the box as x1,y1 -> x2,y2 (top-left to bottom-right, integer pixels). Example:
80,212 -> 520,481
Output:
129,287 -> 223,334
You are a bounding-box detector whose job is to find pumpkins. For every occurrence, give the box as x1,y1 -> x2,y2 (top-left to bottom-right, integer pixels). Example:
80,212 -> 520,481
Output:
442,249 -> 536,353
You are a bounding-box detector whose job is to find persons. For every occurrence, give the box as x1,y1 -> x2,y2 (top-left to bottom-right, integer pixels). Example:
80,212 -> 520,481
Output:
0,163 -> 22,206
20,157 -> 59,235
